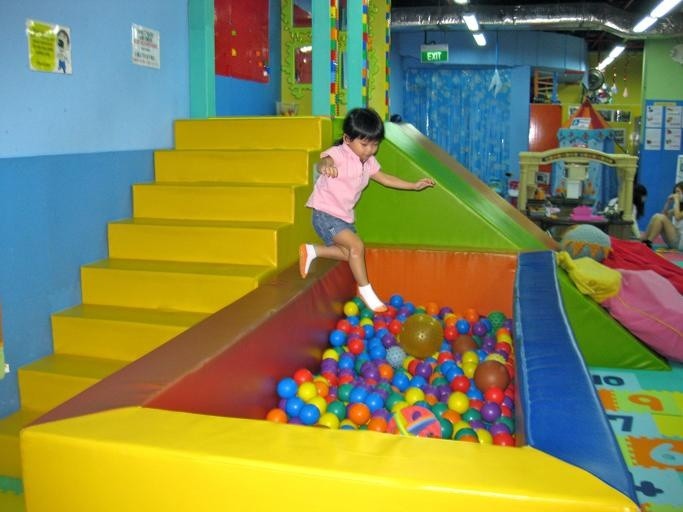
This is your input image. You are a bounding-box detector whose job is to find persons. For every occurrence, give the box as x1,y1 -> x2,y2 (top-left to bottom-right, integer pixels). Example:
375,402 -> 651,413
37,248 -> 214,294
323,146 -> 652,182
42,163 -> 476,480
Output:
618,184 -> 648,242
296,105 -> 434,312
647,181 -> 683,250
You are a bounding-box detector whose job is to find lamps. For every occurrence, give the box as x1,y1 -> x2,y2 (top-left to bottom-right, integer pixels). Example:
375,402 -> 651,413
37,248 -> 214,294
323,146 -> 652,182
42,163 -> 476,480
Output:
453,0 -> 487,48
594,1 -> 682,73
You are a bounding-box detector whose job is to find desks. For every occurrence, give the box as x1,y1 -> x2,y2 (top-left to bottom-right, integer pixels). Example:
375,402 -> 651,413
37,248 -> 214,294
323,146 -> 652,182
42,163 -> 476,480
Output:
528,207 -> 633,242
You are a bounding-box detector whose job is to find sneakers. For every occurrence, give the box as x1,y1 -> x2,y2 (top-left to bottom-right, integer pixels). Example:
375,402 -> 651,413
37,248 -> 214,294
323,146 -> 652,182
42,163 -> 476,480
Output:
358,290 -> 388,313
299,242 -> 308,280
